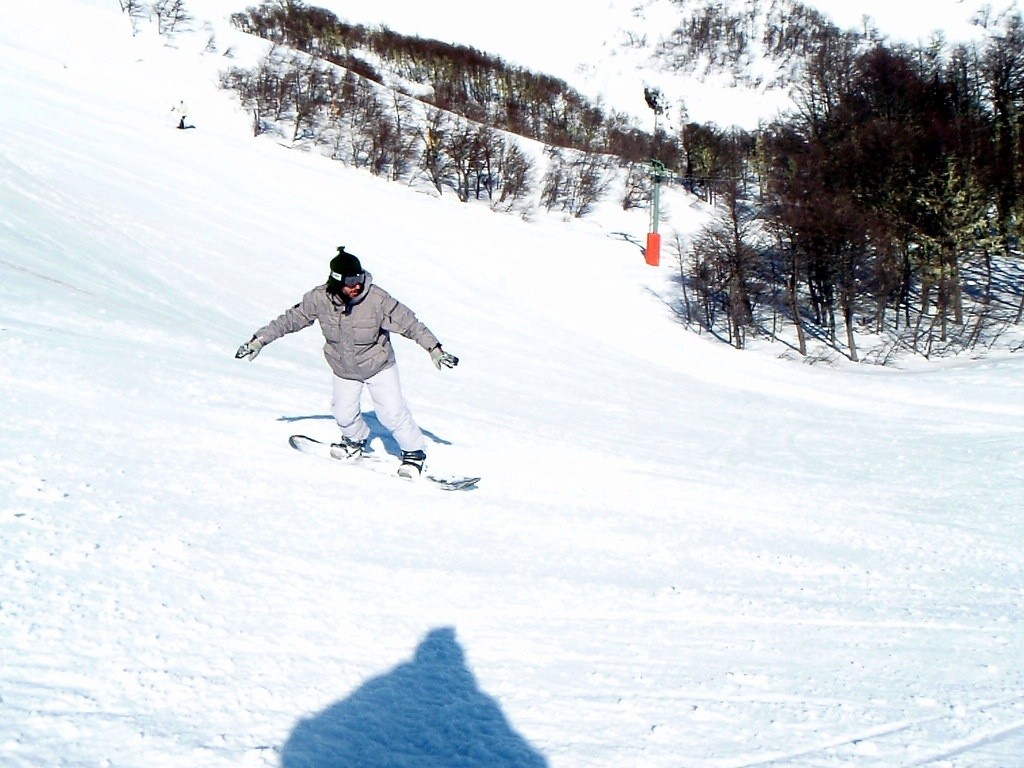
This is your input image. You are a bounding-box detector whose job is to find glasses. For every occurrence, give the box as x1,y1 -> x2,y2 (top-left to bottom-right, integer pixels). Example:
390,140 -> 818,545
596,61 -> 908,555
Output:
345,274 -> 365,286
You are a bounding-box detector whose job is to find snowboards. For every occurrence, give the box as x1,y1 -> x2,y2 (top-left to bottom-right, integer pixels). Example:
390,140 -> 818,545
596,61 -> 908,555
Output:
290,433 -> 482,490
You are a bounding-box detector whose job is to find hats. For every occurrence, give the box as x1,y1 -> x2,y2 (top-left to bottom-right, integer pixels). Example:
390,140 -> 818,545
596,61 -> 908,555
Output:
326,246 -> 362,295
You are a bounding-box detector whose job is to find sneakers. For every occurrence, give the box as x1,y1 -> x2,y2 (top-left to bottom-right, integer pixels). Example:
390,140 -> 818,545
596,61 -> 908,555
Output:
398,450 -> 427,479
340,436 -> 367,459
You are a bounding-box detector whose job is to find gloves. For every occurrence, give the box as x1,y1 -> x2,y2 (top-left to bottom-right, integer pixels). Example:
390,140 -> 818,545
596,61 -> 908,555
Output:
235,338 -> 263,361
430,346 -> 459,371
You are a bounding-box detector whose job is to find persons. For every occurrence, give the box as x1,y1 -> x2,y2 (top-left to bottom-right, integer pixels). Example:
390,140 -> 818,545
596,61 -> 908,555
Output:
174,99 -> 187,128
234,246 -> 459,478
